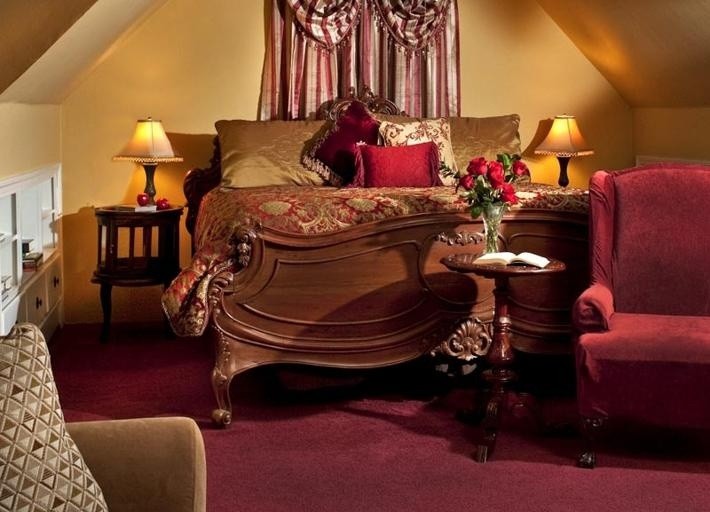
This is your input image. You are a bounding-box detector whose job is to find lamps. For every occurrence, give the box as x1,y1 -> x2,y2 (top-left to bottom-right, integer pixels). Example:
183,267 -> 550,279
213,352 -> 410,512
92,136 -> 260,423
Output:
534,114 -> 595,189
113,115 -> 185,208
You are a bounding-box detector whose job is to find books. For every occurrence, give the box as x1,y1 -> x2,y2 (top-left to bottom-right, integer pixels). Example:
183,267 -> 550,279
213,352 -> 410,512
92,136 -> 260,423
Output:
135,206 -> 157,212
472,252 -> 550,269
22,237 -> 44,272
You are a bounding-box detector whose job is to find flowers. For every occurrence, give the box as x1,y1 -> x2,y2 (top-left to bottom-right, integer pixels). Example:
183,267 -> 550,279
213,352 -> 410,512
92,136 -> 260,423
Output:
457,152 -> 532,222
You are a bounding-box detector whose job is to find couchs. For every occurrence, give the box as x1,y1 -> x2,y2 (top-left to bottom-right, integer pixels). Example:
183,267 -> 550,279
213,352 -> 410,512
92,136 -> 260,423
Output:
55,415 -> 207,510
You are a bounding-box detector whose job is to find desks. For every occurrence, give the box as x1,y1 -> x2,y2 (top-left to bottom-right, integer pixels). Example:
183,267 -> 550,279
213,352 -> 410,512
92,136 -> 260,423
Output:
91,204 -> 186,341
440,253 -> 568,466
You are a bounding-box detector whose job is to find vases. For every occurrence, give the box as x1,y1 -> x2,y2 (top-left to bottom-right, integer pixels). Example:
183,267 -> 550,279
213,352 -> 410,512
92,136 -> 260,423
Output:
481,196 -> 503,254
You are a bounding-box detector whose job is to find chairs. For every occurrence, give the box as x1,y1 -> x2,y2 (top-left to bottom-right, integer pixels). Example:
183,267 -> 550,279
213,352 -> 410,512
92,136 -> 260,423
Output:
580,167 -> 708,471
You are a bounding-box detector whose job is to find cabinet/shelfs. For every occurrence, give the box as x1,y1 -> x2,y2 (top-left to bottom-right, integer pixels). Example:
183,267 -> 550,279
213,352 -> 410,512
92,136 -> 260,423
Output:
1,160 -> 64,346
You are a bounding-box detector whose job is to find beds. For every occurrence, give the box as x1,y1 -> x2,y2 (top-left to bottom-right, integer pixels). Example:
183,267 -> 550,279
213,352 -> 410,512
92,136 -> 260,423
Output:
201,85 -> 544,430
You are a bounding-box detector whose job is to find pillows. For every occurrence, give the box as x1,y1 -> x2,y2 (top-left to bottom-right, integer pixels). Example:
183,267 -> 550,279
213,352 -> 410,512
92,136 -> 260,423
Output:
384,114 -> 524,173
371,118 -> 459,188
0,324 -> 107,512
356,143 -> 439,191
216,118 -> 333,189
300,100 -> 380,185
